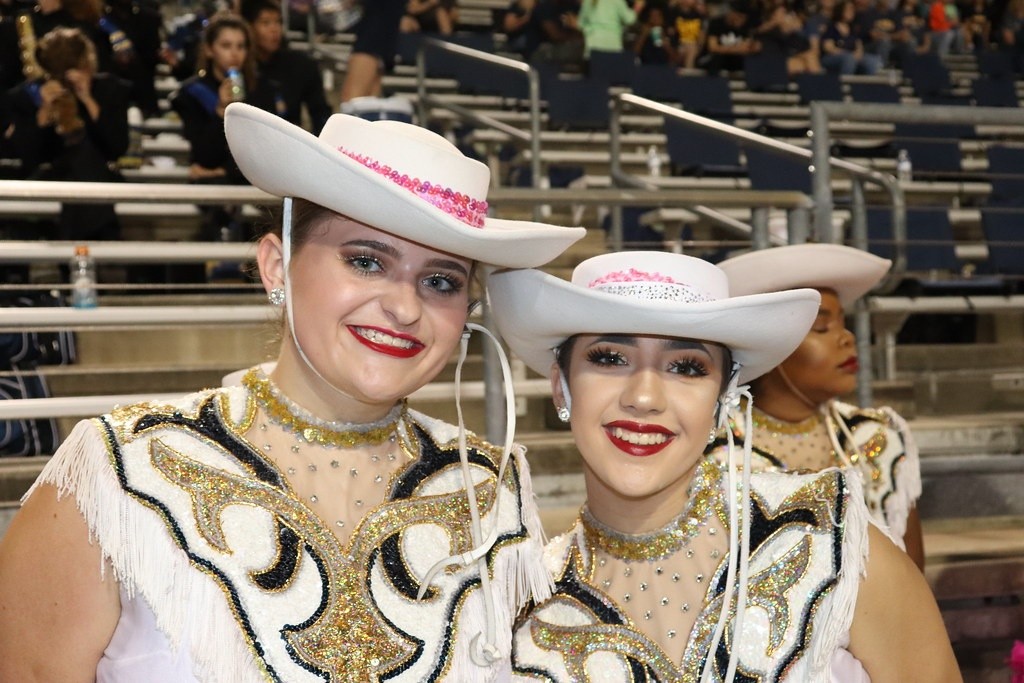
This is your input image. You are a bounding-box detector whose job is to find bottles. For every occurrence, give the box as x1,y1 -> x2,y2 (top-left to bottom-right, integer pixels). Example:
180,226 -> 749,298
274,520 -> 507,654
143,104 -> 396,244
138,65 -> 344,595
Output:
898,148 -> 912,186
68,244 -> 97,309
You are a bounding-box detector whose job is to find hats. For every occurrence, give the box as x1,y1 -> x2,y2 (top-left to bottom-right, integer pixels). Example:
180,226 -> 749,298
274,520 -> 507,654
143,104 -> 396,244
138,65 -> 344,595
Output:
223,96 -> 587,270
715,243 -> 891,309
488,250 -> 822,385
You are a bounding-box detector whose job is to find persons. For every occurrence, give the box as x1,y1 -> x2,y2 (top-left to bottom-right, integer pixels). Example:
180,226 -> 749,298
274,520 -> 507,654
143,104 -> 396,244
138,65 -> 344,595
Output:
719,242 -> 927,569
1,0 -> 1024,282
0,104 -> 545,683
488,252 -> 967,683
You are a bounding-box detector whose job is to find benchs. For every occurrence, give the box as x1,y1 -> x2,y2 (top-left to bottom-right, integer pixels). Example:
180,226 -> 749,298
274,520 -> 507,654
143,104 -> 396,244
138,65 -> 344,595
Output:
0,0 -> 1024,589
